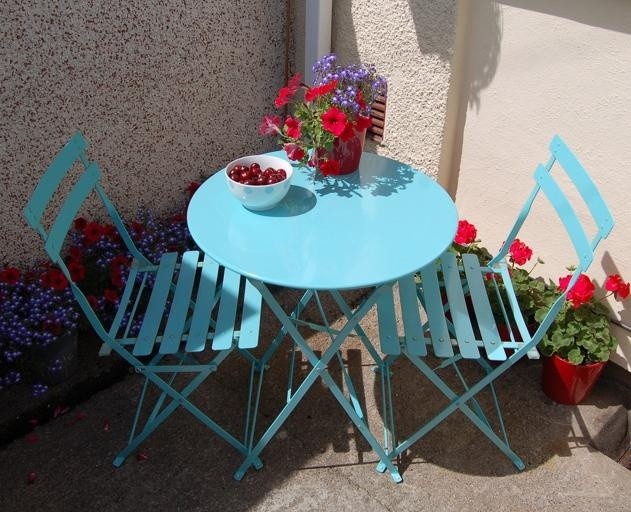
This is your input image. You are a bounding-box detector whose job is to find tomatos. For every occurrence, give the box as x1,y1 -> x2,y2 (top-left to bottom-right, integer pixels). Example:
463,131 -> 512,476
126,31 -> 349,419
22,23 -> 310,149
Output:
230,163 -> 286,185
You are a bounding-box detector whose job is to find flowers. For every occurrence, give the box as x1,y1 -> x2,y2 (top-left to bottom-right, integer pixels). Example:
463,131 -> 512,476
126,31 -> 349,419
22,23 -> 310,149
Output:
413,212 -> 630,362
258,51 -> 387,178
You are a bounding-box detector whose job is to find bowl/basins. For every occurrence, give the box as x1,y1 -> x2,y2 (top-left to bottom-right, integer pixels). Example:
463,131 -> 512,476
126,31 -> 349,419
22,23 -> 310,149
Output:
225,155 -> 296,212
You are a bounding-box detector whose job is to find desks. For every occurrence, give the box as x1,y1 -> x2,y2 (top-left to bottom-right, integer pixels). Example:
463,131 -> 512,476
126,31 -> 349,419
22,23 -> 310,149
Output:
186,146 -> 462,484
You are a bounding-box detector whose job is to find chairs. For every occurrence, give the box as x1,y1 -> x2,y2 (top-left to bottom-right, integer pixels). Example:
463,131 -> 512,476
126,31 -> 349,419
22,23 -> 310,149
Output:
377,136 -> 615,472
26,126 -> 262,471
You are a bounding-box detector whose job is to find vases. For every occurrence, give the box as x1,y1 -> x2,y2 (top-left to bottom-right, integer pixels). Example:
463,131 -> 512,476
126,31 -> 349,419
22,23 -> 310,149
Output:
540,352 -> 607,406
322,125 -> 367,173
